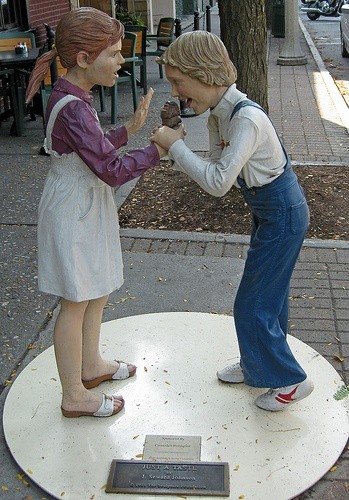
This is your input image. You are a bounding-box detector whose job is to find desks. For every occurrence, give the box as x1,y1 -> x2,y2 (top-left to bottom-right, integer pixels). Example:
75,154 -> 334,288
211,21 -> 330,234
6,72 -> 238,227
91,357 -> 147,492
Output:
0,47 -> 45,138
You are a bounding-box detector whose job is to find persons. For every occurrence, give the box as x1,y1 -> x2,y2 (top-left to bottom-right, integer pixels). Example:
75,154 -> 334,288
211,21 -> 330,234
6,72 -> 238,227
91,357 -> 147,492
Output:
148,31 -> 314,411
25,7 -> 186,419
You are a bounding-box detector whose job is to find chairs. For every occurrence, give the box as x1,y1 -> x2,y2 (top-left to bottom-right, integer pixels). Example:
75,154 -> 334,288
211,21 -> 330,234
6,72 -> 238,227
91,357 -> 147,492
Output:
0,18 -> 175,137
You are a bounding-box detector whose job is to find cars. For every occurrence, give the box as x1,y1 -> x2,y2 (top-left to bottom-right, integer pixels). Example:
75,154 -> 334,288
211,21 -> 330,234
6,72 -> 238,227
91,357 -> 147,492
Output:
340,0 -> 349,58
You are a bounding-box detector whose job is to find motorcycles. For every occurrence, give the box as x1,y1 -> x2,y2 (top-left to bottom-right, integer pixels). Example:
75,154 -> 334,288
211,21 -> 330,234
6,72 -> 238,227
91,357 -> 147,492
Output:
301,0 -> 349,21
